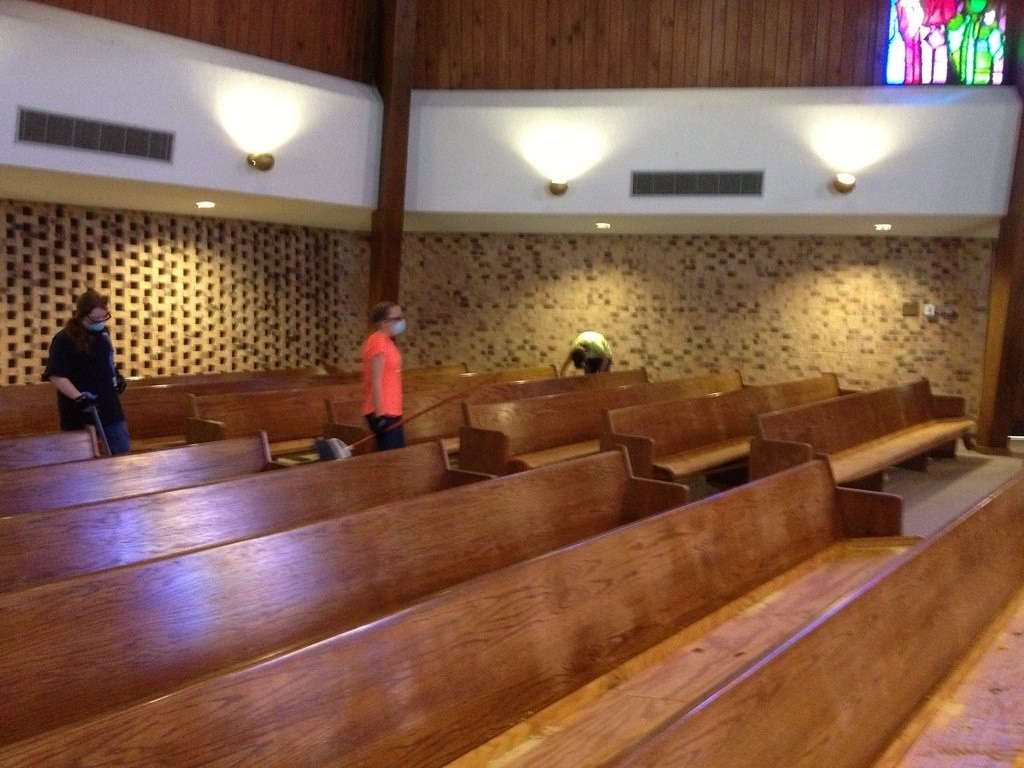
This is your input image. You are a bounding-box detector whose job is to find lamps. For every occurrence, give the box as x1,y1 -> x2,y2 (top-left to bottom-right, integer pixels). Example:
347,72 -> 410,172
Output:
548,183 -> 568,194
834,175 -> 856,193
247,152 -> 275,171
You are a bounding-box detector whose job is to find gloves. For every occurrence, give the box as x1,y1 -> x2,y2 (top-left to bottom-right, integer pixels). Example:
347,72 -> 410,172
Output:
115,375 -> 128,394
76,393 -> 99,412
373,416 -> 393,435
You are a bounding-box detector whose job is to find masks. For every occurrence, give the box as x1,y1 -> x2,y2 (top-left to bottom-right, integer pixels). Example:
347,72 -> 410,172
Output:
385,319 -> 406,336
84,318 -> 106,331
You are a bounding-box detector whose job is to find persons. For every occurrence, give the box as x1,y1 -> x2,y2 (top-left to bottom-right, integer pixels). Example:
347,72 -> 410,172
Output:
560,331 -> 614,377
46,290 -> 130,455
360,301 -> 407,451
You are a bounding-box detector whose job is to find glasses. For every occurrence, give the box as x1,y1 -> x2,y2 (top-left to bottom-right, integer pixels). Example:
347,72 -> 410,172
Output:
87,311 -> 111,324
381,314 -> 407,322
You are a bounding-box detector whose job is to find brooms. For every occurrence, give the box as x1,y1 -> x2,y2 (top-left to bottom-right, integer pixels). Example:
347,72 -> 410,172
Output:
312,382 -> 484,463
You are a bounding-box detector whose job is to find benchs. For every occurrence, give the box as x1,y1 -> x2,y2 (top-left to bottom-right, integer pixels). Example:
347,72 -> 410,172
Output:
0,357 -> 1024,765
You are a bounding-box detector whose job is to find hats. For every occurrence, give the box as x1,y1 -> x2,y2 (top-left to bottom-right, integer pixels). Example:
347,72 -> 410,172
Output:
572,347 -> 587,369
